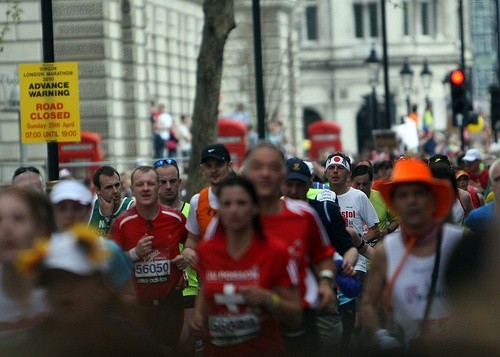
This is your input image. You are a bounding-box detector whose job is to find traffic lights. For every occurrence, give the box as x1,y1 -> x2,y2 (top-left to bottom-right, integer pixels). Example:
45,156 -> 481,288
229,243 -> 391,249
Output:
448,69 -> 469,127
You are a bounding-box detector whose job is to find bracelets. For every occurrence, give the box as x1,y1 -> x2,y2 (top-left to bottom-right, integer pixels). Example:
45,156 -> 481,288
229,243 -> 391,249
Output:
128,248 -> 140,262
356,239 -> 365,250
386,228 -> 392,233
318,269 -> 335,282
267,294 -> 280,312
104,215 -> 115,225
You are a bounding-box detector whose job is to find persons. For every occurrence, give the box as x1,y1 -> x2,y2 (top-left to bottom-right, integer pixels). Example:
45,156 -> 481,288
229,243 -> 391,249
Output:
0,101 -> 500,357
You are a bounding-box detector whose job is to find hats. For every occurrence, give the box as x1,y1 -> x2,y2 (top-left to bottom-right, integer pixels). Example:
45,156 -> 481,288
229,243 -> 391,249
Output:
379,158 -> 453,223
287,158 -> 312,184
202,144 -> 231,163
455,170 -> 470,180
50,180 -> 93,206
40,231 -> 107,275
462,148 -> 481,162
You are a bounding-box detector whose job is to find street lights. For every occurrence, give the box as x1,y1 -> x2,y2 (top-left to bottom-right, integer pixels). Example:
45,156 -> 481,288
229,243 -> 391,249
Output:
363,43 -> 435,129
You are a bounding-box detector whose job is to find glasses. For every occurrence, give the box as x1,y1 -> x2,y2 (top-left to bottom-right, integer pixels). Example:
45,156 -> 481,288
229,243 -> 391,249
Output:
154,159 -> 179,179
493,176 -> 500,182
12,166 -> 40,180
